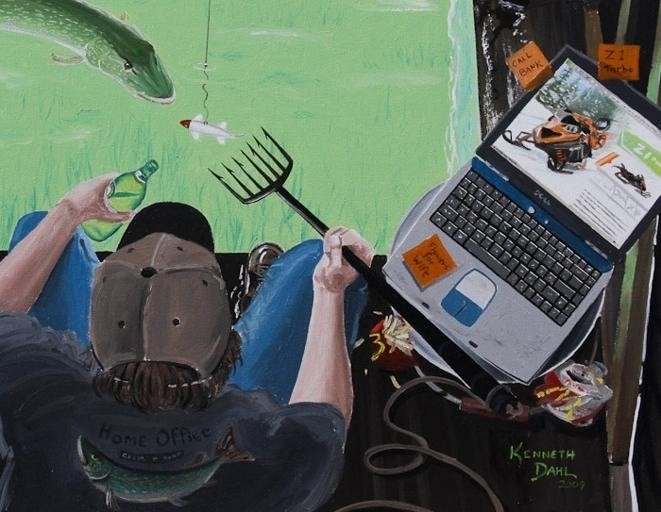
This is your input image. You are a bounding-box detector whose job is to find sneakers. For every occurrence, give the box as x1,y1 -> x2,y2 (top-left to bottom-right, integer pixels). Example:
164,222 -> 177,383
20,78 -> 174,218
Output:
242,244 -> 284,312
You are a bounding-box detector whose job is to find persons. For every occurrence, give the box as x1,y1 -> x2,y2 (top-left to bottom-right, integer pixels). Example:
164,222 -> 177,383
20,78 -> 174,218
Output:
0,172 -> 375,512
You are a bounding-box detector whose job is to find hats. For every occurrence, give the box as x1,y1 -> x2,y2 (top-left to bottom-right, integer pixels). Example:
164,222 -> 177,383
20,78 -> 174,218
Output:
86,202 -> 232,389
